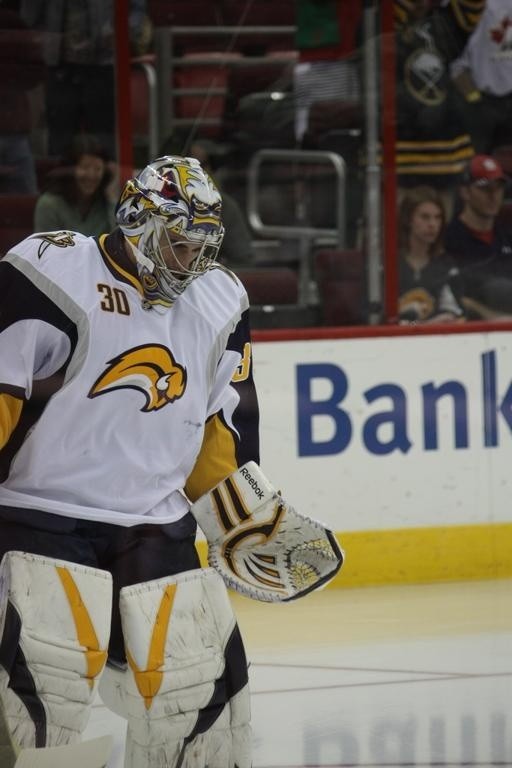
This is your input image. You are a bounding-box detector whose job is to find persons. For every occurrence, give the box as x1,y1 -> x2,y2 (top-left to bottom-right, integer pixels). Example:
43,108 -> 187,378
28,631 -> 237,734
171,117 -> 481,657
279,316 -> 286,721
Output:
1,1 -> 512,331
5,151 -> 342,768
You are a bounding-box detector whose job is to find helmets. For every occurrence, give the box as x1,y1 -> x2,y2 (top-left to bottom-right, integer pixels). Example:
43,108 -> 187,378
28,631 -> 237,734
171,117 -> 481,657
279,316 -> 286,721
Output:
114,155 -> 225,300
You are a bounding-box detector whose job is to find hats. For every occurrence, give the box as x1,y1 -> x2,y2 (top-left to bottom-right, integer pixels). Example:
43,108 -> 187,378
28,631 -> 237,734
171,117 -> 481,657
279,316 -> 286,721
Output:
464,156 -> 508,186
